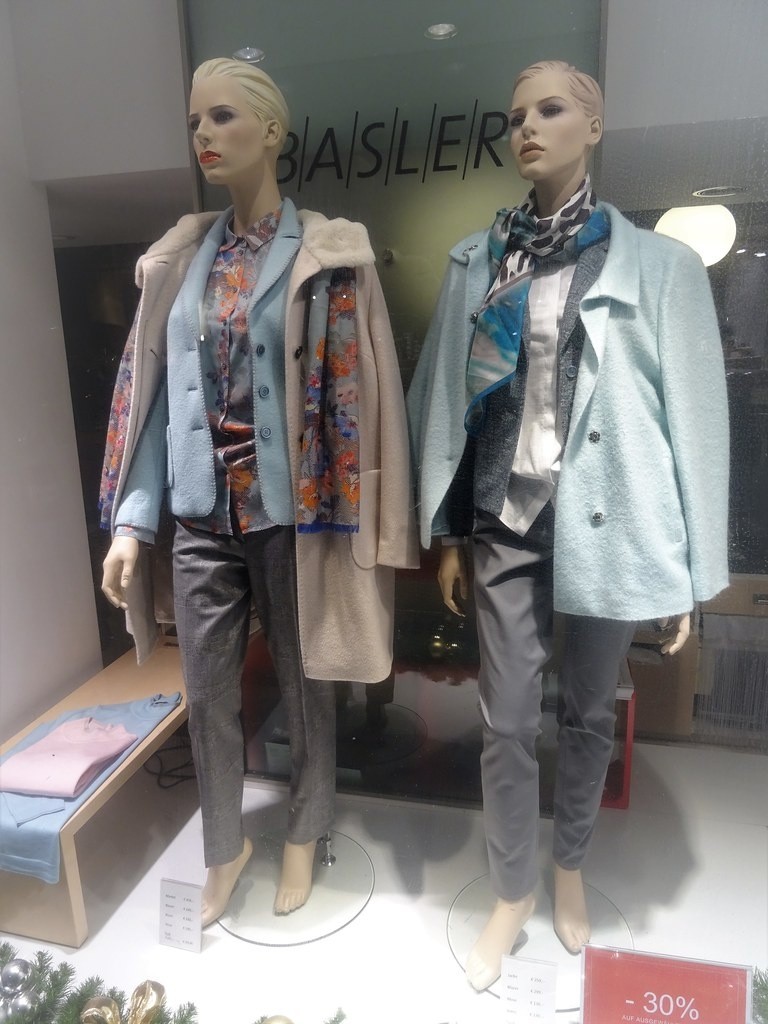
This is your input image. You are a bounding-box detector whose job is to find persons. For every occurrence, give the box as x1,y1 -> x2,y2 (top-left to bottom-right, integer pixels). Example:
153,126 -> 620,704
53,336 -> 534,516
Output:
405,61 -> 730,990
97,57 -> 421,929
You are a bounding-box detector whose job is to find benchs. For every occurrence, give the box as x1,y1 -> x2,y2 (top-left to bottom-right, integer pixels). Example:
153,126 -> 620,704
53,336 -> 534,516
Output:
0,645 -> 190,948
241,632 -> 637,810
